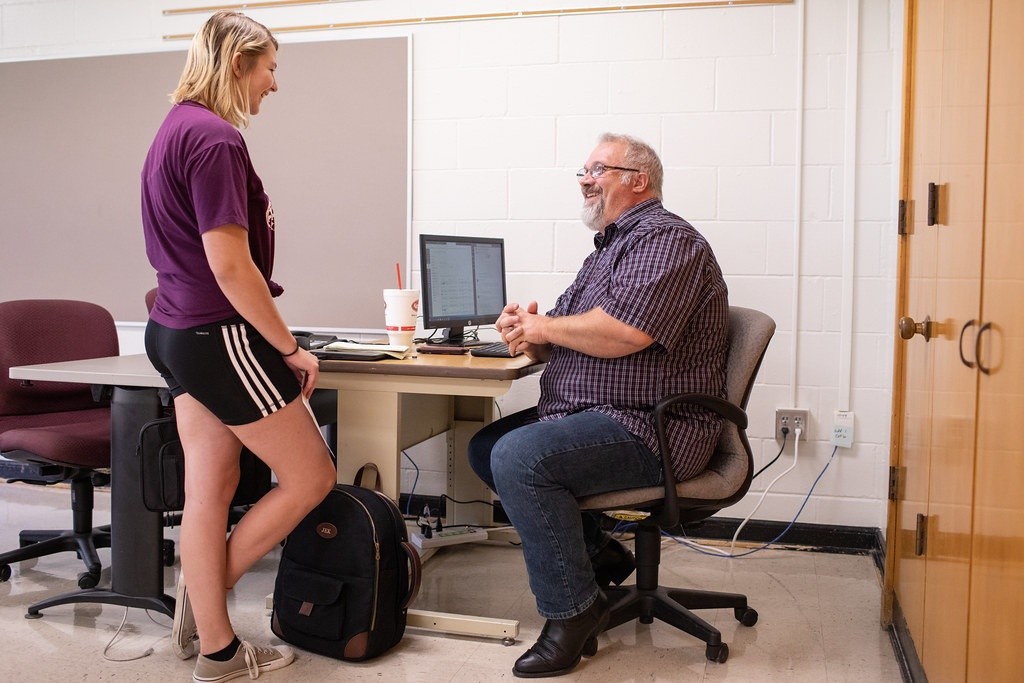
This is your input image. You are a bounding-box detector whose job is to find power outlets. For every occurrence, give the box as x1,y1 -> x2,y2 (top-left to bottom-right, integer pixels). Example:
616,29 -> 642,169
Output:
774,409 -> 811,442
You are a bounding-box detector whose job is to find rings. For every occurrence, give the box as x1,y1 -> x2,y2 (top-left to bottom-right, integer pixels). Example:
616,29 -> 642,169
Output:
509,325 -> 514,331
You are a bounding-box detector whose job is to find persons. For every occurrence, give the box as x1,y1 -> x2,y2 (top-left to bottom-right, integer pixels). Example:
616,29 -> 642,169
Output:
466,133 -> 735,679
139,12 -> 333,683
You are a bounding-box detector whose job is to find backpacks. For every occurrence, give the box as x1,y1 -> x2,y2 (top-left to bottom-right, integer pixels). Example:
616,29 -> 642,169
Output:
270,463 -> 421,663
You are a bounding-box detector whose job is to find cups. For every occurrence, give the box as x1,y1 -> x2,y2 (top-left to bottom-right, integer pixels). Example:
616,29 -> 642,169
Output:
383,288 -> 420,353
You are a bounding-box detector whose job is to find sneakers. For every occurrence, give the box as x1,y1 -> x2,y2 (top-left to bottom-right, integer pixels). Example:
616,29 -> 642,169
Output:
171,568 -> 195,659
193,636 -> 294,683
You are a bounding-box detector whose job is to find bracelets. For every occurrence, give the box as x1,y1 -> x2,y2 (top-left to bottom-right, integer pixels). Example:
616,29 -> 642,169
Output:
283,340 -> 299,356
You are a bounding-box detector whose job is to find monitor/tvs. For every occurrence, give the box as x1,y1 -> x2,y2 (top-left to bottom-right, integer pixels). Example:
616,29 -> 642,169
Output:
418,234 -> 507,346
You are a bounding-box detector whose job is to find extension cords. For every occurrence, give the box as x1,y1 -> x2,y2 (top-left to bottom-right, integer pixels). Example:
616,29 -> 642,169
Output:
411,526 -> 488,548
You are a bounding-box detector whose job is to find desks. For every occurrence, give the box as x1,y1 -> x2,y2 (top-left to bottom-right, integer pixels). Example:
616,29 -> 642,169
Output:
10,334 -> 545,642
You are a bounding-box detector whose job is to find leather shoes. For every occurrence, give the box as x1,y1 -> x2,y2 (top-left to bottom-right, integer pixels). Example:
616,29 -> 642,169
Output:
592,539 -> 636,589
512,589 -> 610,679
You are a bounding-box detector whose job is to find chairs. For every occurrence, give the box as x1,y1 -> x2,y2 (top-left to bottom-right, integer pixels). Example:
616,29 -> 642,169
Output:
0,300 -> 176,591
566,303 -> 780,662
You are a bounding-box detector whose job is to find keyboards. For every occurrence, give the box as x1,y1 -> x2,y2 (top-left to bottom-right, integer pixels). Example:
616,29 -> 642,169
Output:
471,341 -> 524,357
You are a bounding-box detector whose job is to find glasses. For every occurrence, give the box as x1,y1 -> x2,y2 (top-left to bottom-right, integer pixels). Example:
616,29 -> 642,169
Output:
576,163 -> 640,182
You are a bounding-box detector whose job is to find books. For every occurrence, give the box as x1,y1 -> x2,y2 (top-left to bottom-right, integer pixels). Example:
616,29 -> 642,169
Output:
323,341 -> 410,360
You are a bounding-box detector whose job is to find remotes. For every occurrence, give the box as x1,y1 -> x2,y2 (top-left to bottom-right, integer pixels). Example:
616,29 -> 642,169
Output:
416,345 -> 469,355
